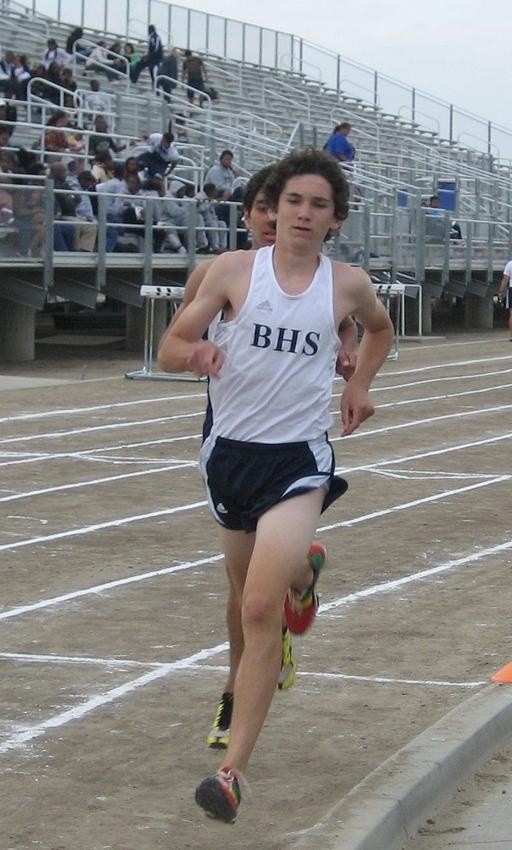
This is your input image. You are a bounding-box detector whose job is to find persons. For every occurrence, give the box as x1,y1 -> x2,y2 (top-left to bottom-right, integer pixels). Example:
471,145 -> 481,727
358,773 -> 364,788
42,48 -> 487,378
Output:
156,161 -> 364,752
155,147 -> 397,826
495,259 -> 512,342
426,195 -> 462,242
0,20 -> 263,255
322,121 -> 362,210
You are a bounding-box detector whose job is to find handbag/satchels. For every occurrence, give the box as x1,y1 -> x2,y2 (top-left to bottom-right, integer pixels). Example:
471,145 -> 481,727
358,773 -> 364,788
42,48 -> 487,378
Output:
202,87 -> 218,101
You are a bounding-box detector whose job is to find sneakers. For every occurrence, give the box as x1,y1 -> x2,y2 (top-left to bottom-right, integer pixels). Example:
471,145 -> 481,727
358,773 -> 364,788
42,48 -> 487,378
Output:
194,767 -> 241,825
284,543 -> 326,635
277,629 -> 296,690
206,691 -> 233,749
